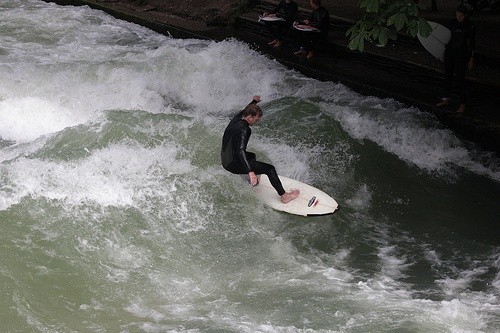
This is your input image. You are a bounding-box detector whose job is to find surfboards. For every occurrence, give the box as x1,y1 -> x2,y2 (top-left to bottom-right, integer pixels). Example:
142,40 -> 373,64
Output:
416,20 -> 452,62
253,174 -> 338,216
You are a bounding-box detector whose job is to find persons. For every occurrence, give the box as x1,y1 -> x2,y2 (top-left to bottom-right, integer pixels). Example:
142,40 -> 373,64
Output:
293,0 -> 330,59
263,0 -> 298,47
221,95 -> 300,204
435,4 -> 475,113
